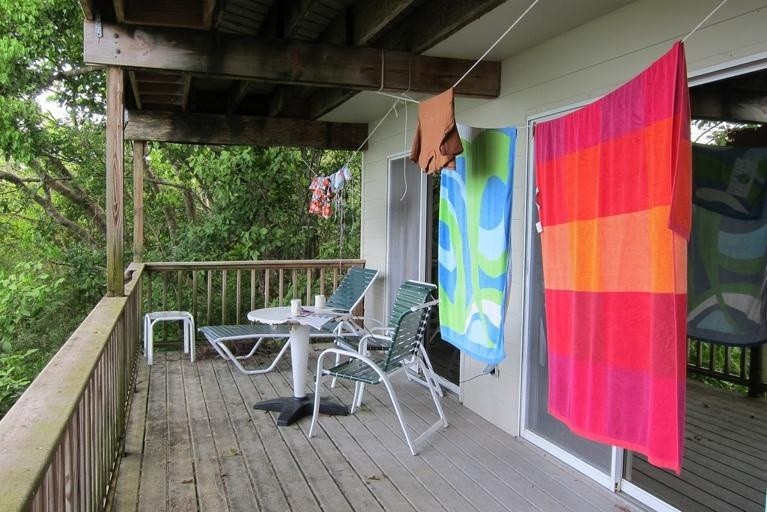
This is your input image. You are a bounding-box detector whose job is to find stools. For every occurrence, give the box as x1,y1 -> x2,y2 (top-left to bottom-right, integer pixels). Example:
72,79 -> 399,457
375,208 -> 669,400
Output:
142,311 -> 196,364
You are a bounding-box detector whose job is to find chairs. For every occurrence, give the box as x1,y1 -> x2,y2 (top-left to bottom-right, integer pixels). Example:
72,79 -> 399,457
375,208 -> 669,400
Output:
303,295 -> 452,460
324,279 -> 437,416
192,261 -> 385,377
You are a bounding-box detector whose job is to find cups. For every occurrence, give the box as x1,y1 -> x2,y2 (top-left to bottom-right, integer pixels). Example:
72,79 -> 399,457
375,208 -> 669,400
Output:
314,294 -> 326,308
290,299 -> 302,314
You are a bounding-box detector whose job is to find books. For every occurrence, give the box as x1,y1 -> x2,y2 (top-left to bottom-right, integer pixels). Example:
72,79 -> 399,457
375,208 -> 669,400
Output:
287,312 -> 341,330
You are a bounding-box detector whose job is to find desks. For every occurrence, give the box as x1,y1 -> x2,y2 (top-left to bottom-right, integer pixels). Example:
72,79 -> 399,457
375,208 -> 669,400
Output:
245,299 -> 353,431
